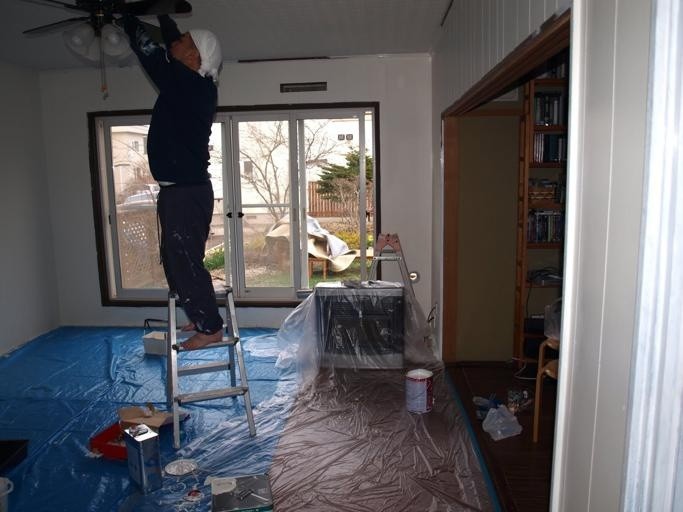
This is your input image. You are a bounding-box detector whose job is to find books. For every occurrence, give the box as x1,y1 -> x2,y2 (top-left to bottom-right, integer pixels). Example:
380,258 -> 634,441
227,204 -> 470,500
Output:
527,60 -> 566,244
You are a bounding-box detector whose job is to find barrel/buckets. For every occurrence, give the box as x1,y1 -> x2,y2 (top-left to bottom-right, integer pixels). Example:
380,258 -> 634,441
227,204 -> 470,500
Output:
0,476 -> 15,512
405,368 -> 435,415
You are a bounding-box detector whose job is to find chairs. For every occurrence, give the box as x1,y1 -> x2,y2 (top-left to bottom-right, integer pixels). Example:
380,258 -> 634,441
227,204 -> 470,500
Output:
534,299 -> 560,445
309,237 -> 329,280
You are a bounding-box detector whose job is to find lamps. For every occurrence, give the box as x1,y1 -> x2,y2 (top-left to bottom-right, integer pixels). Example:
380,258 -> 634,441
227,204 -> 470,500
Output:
65,22 -> 135,65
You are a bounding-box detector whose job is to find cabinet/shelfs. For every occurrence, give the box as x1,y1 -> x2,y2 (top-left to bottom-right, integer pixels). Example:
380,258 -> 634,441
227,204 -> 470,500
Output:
515,70 -> 570,375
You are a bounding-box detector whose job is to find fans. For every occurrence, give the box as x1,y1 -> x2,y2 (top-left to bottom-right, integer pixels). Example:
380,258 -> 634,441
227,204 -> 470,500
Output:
21,0 -> 193,34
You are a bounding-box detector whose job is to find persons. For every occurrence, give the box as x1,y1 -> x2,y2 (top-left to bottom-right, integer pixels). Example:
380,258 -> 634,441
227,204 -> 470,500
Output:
112,12 -> 223,351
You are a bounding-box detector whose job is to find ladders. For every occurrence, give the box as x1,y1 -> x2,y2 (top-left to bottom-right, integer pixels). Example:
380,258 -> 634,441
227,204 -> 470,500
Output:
166,286 -> 257,452
369,232 -> 425,328
